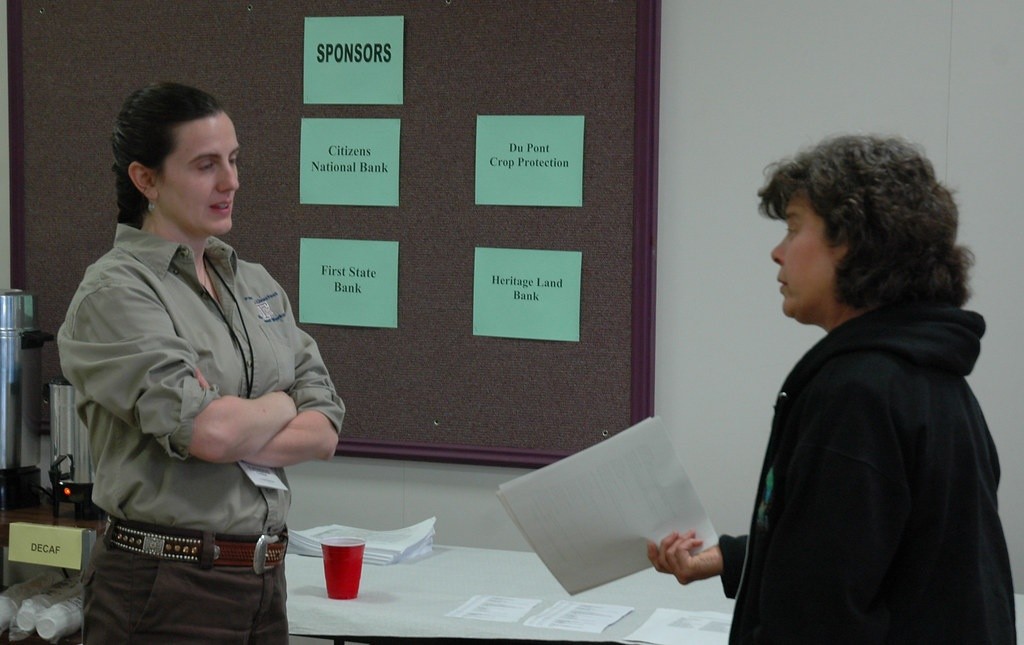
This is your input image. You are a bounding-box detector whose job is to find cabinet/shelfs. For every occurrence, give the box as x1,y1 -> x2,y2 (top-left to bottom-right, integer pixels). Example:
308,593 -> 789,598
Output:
0,492 -> 108,645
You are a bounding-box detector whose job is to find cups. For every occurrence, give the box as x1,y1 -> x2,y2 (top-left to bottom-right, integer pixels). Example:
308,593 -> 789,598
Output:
320,538 -> 366,600
0,570 -> 83,641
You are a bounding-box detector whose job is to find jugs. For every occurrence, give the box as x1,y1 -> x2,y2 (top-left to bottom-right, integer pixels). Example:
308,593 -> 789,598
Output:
42,376 -> 97,504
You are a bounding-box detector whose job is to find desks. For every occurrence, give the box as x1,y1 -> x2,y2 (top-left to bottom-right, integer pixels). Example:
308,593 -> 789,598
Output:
276,545 -> 737,645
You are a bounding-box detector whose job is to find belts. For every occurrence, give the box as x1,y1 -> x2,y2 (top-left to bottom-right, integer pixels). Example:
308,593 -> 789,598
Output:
104,516 -> 288,575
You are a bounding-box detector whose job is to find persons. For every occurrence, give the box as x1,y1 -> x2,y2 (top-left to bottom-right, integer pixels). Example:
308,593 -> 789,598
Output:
57,84 -> 345,645
647,136 -> 1017,645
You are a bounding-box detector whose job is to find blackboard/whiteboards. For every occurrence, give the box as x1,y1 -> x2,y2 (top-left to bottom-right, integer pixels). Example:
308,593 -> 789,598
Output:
7,0 -> 665,469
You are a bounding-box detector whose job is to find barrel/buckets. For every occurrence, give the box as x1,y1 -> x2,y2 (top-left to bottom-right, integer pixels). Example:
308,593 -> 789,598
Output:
1,288 -> 56,478
